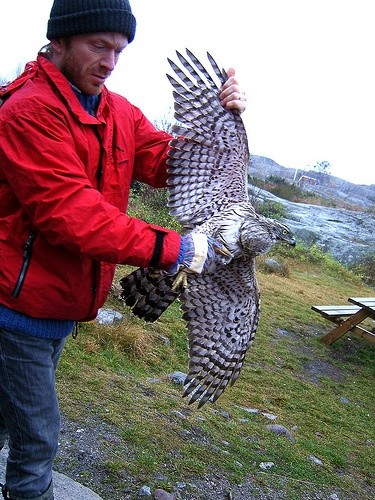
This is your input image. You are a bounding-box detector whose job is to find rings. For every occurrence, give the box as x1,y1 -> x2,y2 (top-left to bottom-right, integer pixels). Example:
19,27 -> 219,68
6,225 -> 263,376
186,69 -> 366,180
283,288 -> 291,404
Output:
238,92 -> 242,100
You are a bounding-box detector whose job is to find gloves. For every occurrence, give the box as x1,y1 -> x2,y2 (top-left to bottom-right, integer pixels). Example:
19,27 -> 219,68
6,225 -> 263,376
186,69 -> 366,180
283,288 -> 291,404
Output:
176,231 -> 234,274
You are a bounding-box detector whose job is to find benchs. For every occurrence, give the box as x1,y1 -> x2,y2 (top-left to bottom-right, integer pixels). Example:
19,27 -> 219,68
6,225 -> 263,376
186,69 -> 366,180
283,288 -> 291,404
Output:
311,305 -> 362,319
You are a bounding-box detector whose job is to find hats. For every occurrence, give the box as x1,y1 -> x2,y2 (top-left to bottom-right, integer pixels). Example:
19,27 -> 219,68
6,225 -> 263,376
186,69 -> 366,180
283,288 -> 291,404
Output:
47,0 -> 136,43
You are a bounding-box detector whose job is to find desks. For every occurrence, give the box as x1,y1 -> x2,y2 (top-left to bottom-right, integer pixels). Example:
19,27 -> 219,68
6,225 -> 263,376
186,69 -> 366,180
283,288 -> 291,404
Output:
320,298 -> 375,348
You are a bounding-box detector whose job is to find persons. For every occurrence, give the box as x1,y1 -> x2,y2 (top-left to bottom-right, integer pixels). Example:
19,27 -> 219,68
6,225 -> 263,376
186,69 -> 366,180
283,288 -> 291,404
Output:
0,0 -> 247,500
